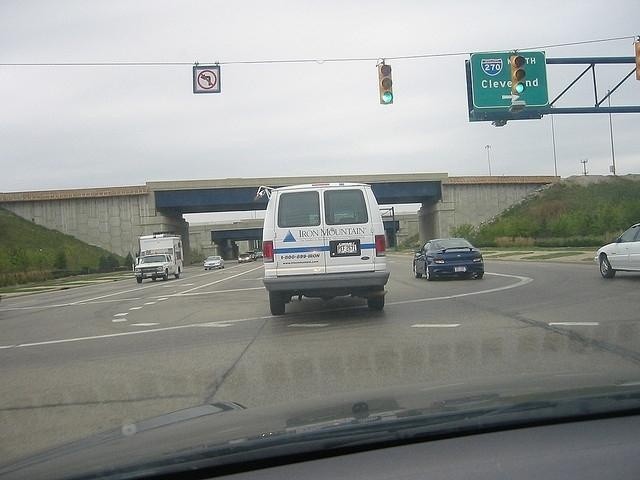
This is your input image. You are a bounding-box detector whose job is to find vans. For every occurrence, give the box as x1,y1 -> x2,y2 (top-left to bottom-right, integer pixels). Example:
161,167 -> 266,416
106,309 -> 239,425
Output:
260,182 -> 392,317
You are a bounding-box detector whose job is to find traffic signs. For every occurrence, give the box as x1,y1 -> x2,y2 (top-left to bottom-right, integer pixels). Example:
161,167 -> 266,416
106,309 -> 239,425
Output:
470,50 -> 549,113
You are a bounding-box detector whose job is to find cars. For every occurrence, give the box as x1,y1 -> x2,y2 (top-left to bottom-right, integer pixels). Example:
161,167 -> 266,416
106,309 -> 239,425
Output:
238,248 -> 263,264
203,256 -> 225,271
594,223 -> 640,278
412,236 -> 484,281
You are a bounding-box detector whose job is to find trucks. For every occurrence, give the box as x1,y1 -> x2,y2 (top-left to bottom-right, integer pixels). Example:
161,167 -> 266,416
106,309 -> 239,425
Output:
132,234 -> 184,284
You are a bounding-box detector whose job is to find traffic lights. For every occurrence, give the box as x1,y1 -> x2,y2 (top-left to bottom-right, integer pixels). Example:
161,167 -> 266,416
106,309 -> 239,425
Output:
378,62 -> 392,104
510,56 -> 527,97
